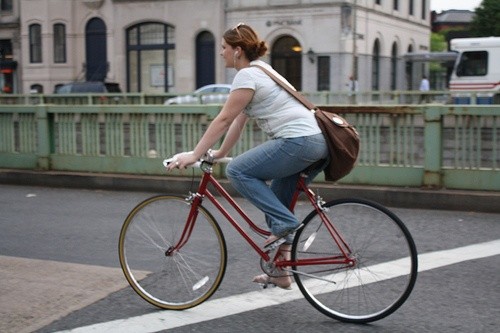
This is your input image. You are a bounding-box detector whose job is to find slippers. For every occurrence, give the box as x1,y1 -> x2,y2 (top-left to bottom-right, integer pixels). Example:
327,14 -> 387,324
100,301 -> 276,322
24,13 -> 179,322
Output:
252,273 -> 292,292
261,222 -> 306,250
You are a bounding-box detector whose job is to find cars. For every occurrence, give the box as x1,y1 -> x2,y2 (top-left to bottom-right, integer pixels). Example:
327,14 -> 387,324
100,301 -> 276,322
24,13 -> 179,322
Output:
53,82 -> 122,95
162,83 -> 232,106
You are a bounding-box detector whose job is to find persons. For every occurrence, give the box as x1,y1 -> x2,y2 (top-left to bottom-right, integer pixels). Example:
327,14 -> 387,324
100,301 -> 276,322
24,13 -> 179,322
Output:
419,75 -> 430,103
164,24 -> 329,288
346,77 -> 359,96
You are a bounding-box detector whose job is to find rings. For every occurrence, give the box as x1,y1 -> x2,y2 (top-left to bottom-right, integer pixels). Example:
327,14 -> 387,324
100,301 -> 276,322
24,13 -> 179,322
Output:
175,162 -> 180,167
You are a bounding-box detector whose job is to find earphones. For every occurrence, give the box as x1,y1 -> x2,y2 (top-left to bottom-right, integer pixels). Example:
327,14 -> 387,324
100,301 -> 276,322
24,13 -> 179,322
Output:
235,51 -> 238,54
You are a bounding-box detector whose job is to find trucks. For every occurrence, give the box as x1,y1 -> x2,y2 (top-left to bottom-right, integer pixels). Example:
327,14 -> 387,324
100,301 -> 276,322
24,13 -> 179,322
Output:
450,37 -> 500,105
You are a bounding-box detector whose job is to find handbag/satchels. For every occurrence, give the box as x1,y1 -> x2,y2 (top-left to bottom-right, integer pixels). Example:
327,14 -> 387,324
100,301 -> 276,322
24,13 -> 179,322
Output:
314,105 -> 361,181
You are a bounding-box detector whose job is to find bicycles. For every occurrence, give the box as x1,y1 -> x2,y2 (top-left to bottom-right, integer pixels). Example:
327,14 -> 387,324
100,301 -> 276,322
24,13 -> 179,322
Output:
118,153 -> 418,323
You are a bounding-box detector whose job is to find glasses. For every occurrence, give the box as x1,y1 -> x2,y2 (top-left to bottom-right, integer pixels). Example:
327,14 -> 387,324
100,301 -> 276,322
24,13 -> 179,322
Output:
233,23 -> 247,40
